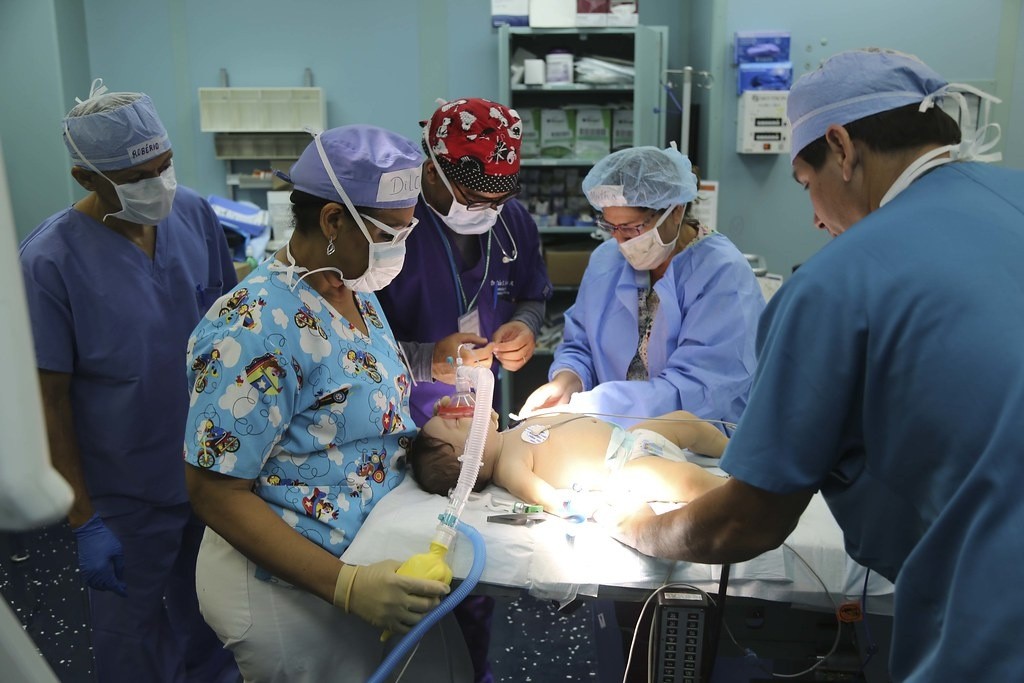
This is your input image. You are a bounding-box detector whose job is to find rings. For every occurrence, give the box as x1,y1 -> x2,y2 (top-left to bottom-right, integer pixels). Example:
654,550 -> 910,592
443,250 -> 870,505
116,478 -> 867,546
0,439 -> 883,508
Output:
523,356 -> 526,365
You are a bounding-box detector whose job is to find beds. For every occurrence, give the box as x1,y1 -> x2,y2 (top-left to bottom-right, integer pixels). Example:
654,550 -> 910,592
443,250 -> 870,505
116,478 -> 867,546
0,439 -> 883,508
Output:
333,448 -> 894,683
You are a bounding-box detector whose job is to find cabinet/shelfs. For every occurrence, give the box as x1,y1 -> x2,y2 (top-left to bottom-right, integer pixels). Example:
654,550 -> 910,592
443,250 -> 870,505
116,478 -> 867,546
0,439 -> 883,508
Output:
500,21 -> 669,426
197,66 -> 327,202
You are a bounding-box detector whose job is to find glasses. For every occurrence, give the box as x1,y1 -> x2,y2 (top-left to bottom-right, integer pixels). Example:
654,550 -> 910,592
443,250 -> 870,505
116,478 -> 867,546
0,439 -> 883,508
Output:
597,210 -> 659,236
361,212 -> 420,246
451,178 -> 521,211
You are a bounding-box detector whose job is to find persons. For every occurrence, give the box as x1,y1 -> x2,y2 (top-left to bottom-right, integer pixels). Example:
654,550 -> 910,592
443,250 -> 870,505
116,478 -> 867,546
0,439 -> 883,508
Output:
184,127 -> 499,683
519,145 -> 766,438
410,405 -> 731,519
373,97 -> 553,683
591,47 -> 1024,683
16,91 -> 240,683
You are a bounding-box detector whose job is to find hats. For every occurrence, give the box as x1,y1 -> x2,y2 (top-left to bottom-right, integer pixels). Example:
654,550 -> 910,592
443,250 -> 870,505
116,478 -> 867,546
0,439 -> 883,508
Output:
290,125 -> 422,209
788,47 -> 950,166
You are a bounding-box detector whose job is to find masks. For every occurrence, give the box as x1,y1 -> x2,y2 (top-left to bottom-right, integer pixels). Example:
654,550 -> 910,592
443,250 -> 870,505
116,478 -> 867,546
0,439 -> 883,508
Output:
342,240 -> 406,293
442,199 -> 504,235
619,226 -> 676,271
109,159 -> 177,225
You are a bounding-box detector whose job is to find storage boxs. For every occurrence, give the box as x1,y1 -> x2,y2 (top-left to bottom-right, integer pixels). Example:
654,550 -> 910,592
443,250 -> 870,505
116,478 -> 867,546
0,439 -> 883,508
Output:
516,168 -> 597,226
522,57 -> 545,86
577,0 -> 638,13
575,108 -> 611,160
528,0 -> 577,28
613,108 -> 633,152
545,244 -> 592,286
545,51 -> 573,84
516,107 -> 541,159
539,107 -> 575,159
606,12 -> 639,27
490,0 -> 529,26
577,12 -> 606,27
